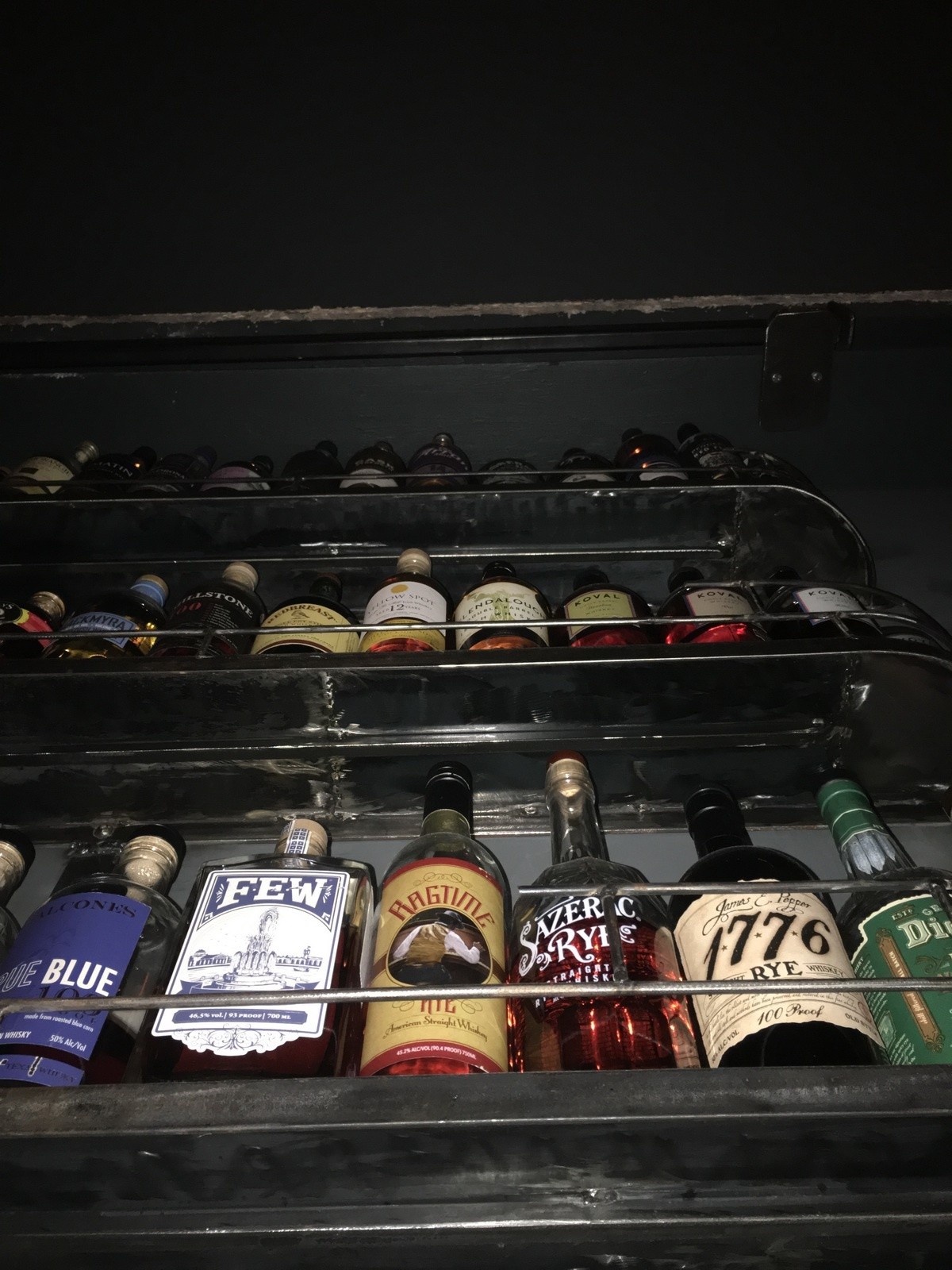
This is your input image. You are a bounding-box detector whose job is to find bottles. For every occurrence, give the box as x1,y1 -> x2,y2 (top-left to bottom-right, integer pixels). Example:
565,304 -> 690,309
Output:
0,757 -> 952,1087
0,423 -> 754,500
0,549 -> 885,672
